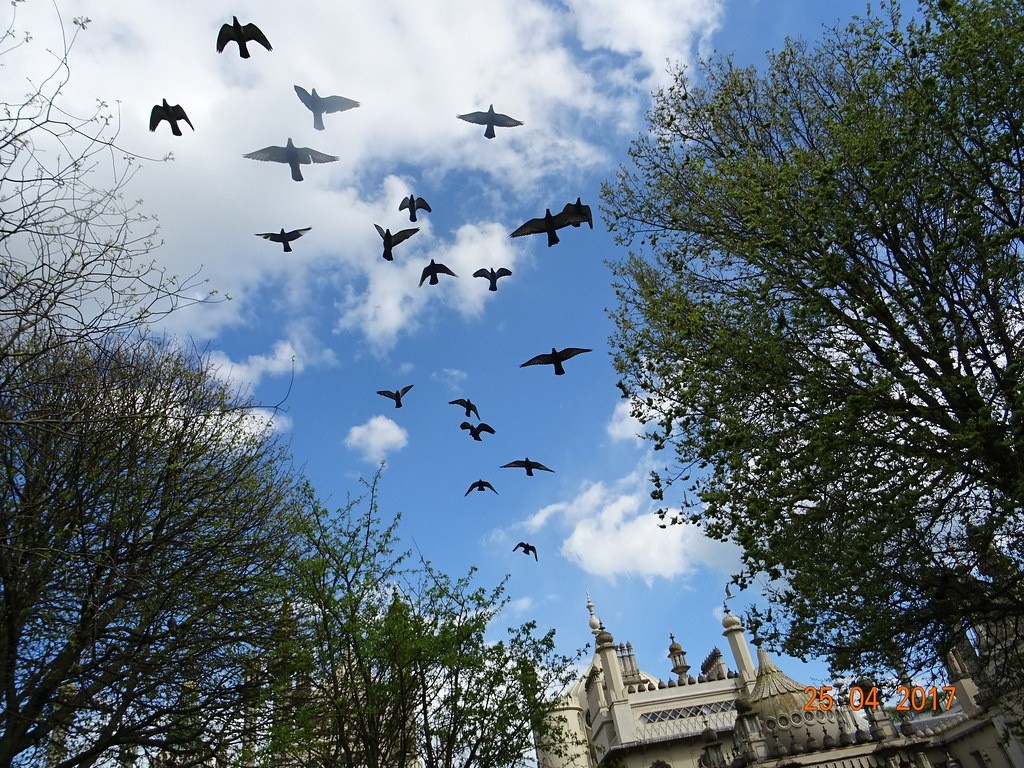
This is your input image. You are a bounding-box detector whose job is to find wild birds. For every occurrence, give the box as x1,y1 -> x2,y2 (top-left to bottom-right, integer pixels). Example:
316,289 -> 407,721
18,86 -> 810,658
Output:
418,259 -> 458,287
473,267 -> 511,291
518,347 -> 592,375
464,479 -> 499,497
509,197 -> 592,248
373,223 -> 420,262
242,138 -> 338,181
295,85 -> 361,131
150,99 -> 195,136
376,385 -> 414,408
500,458 -> 555,476
255,227 -> 313,252
216,16 -> 273,58
460,422 -> 496,442
449,398 -> 481,420
456,104 -> 523,139
399,195 -> 432,222
513,542 -> 538,561
